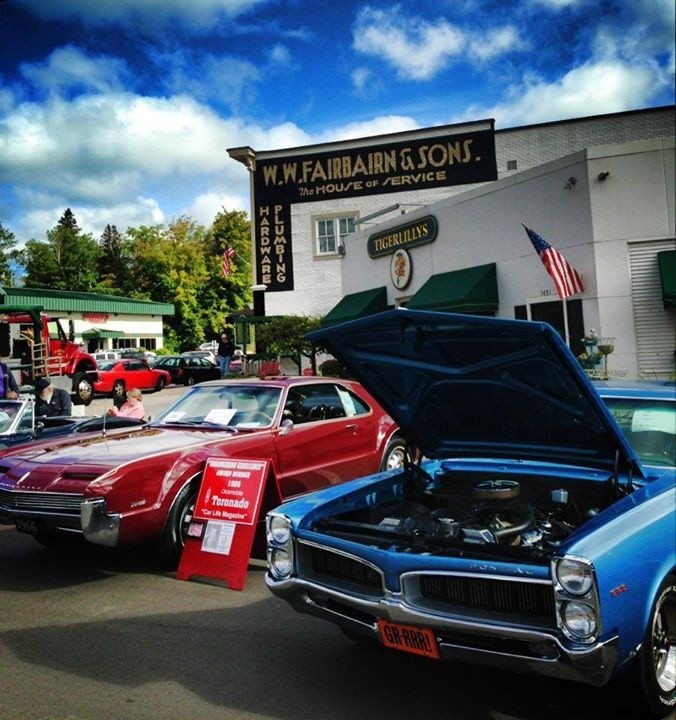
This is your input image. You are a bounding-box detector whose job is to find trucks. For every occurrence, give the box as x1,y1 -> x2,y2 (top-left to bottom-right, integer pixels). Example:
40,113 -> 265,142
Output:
0,306 -> 100,406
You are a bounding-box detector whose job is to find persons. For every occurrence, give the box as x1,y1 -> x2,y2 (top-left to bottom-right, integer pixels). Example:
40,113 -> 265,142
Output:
34,380 -> 72,419
109,388 -> 147,422
215,333 -> 235,377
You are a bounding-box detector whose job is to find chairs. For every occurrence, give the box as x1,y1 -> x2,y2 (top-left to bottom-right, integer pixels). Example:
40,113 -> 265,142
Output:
286,401 -> 340,421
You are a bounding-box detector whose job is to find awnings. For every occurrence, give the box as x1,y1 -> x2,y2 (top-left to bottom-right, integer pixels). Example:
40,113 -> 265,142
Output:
320,286 -> 387,328
82,328 -> 125,339
658,250 -> 676,305
405,262 -> 499,313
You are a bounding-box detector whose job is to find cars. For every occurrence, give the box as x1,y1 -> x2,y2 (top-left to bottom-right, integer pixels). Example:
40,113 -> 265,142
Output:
88,351 -> 172,401
264,308 -> 676,720
180,340 -> 244,371
153,356 -> 222,386
0,377 -> 421,571
0,398 -> 151,456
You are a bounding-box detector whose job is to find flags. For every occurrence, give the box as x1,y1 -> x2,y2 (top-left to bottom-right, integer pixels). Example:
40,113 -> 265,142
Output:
526,227 -> 583,299
221,245 -> 235,275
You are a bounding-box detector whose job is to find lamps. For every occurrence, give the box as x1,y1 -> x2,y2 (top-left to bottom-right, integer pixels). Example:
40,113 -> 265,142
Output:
338,245 -> 345,258
599,171 -> 610,181
565,177 -> 578,191
